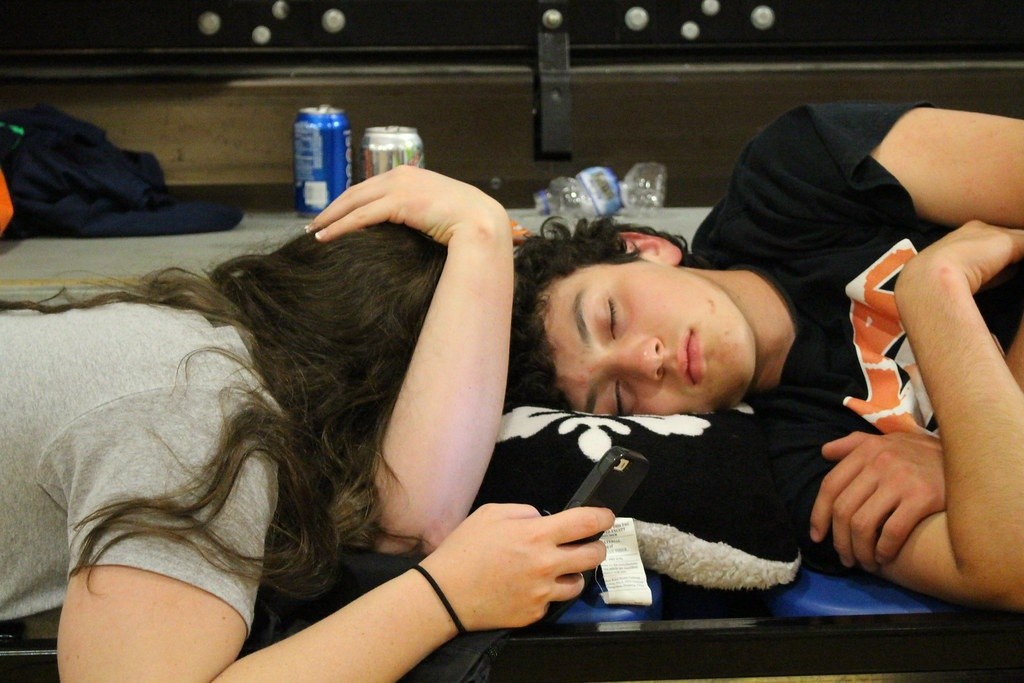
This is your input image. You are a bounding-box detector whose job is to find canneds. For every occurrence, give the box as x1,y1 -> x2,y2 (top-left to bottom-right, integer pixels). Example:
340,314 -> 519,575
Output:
293,104 -> 354,217
358,126 -> 424,183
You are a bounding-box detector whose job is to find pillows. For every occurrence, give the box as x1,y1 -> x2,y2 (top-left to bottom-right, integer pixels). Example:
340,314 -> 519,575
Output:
484,400 -> 804,593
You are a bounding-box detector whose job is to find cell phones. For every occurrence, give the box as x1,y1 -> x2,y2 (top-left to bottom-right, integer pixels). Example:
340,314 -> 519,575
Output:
561,447 -> 650,547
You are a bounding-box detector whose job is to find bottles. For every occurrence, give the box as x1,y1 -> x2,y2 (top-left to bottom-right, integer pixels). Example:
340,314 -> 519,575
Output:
533,161 -> 667,220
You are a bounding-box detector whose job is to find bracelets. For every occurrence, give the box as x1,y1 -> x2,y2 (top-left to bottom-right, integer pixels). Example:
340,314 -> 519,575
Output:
411,564 -> 467,636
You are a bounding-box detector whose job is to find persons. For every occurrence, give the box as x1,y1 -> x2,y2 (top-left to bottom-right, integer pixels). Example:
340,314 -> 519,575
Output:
0,164 -> 615,683
502,102 -> 1024,613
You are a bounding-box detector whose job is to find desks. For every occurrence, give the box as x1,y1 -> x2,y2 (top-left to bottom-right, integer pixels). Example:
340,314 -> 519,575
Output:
0,206 -> 1024,663
